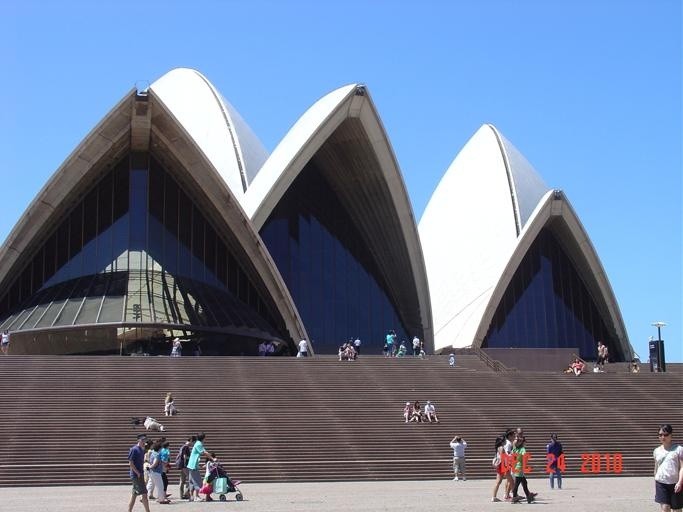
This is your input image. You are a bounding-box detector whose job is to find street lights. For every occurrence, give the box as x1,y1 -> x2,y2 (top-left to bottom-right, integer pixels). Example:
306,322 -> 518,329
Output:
130,302 -> 142,356
648,335 -> 653,371
652,320 -> 667,373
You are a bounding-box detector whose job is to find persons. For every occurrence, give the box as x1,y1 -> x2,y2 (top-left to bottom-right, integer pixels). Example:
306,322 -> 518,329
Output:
338,336 -> 361,362
1,330 -> 11,355
298,338 -> 308,357
383,329 -> 424,358
143,416 -> 166,432
172,338 -> 183,356
653,424 -> 683,512
450,436 -> 468,481
127,433 -> 218,512
448,353 -> 456,368
632,363 -> 640,373
403,400 -> 440,423
492,428 -> 538,505
546,433 -> 562,489
564,341 -> 609,377
266,342 -> 274,356
258,341 -> 267,356
164,392 -> 176,416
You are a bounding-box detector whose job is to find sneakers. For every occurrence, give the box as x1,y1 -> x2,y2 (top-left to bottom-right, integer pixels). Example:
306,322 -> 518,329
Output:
490,490 -> 542,505
139,489 -> 205,505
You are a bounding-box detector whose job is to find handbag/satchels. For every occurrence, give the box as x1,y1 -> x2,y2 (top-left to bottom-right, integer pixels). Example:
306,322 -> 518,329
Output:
491,456 -> 501,468
212,476 -> 228,495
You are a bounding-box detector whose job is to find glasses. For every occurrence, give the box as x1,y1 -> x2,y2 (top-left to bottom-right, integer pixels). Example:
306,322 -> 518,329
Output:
658,433 -> 671,437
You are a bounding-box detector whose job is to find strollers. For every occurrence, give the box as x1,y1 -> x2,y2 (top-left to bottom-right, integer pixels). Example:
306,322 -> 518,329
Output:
201,458 -> 245,502
